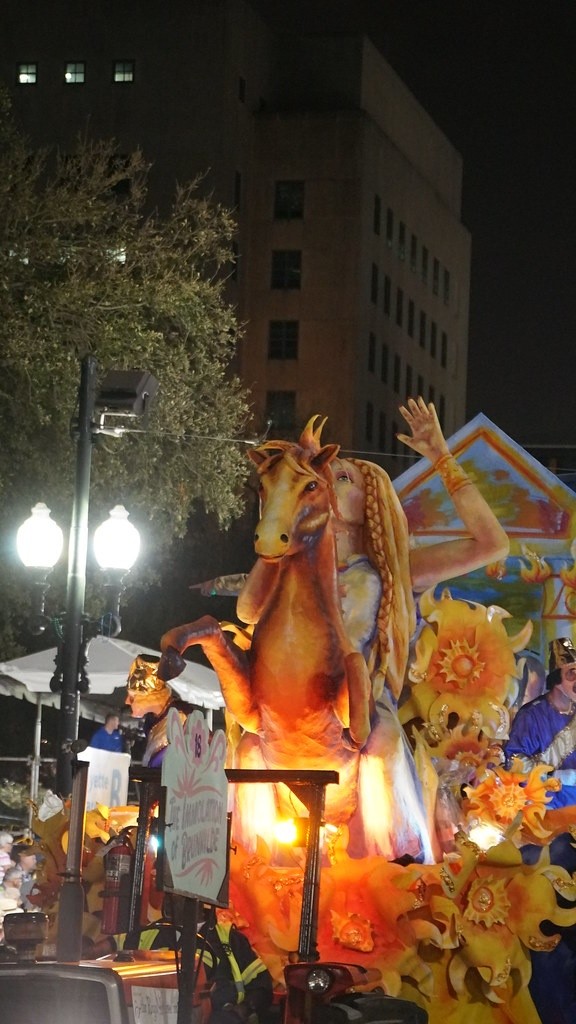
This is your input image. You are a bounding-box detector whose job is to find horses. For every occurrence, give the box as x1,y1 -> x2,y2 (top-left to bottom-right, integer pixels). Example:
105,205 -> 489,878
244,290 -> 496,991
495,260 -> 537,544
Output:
158,444 -> 459,863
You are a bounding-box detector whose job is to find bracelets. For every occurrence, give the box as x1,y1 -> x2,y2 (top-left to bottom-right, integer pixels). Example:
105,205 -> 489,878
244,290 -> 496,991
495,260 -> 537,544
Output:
433,452 -> 475,497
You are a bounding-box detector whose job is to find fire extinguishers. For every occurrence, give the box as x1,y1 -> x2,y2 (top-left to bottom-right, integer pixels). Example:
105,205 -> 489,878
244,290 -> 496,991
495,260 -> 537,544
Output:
94,825 -> 139,935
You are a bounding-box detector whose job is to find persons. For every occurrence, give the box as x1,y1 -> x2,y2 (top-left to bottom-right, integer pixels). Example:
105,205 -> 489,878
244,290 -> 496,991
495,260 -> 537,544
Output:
224,394 -> 513,865
123,893 -> 273,1024
53,738 -> 74,797
0,829 -> 46,942
124,653 -> 215,768
88,713 -> 124,754
503,636 -> 576,879
